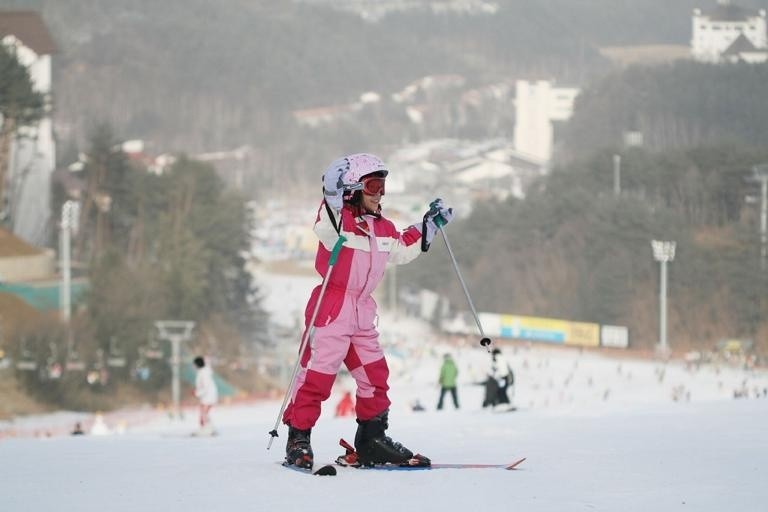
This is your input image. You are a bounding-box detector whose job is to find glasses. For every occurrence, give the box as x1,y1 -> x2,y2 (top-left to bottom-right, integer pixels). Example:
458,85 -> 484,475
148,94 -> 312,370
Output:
342,177 -> 385,195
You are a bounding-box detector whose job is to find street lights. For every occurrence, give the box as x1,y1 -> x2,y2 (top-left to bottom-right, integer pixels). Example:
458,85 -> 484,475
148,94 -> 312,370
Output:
648,238 -> 679,360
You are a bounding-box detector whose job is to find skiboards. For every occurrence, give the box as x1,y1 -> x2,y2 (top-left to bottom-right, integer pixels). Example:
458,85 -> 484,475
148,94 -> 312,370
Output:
273,438 -> 526,477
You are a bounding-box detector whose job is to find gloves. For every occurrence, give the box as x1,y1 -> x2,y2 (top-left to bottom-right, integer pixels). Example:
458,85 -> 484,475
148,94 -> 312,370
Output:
322,159 -> 350,212
412,198 -> 454,245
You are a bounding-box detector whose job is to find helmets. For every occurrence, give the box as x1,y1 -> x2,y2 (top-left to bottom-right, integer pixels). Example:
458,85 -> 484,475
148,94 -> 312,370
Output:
340,153 -> 390,201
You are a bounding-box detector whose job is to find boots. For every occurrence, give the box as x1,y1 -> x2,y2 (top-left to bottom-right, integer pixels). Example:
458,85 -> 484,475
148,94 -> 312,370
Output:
354,409 -> 413,463
286,426 -> 314,467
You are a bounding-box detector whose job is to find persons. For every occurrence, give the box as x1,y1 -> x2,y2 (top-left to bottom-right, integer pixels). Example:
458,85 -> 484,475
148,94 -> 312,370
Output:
434,353 -> 460,409
470,371 -> 496,408
281,154 -> 455,474
191,355 -> 218,434
486,349 -> 515,404
